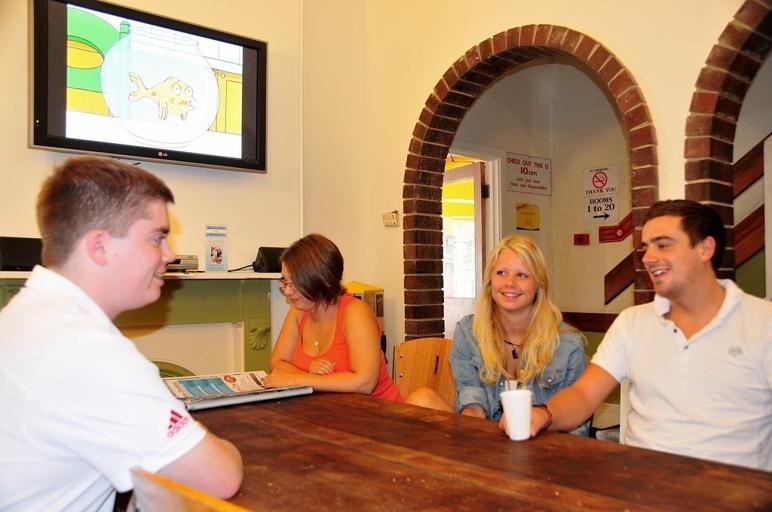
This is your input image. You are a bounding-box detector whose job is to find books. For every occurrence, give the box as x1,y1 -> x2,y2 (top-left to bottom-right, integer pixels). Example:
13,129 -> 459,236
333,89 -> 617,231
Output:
162,368 -> 315,412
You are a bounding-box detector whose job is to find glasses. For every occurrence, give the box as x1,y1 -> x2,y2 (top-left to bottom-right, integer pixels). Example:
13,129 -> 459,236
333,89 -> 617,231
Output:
278,279 -> 296,290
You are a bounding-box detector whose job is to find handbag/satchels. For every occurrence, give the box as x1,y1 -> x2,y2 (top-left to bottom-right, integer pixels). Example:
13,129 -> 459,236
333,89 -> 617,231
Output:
227,247 -> 287,273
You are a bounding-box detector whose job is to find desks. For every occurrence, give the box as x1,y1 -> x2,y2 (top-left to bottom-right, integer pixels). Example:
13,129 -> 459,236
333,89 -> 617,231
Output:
188,393 -> 771,511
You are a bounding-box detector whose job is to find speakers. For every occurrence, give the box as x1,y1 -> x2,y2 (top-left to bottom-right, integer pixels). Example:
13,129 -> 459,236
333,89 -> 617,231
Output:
253,247 -> 286,273
0,236 -> 42,272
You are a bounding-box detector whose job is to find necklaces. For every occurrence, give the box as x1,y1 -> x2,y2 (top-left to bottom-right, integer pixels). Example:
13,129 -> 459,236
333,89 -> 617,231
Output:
307,318 -> 325,346
501,338 -> 524,358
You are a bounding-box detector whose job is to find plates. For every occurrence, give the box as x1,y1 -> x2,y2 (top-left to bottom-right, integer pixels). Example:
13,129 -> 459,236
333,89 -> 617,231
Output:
99,28 -> 221,143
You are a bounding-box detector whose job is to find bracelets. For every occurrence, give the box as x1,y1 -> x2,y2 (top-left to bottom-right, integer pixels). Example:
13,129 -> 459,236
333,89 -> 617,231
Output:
532,404 -> 552,432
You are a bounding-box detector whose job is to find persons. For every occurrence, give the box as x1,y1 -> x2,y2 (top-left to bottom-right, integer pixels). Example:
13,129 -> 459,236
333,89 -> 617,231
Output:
494,197 -> 771,475
405,235 -> 592,439
262,233 -> 406,404
1,152 -> 245,512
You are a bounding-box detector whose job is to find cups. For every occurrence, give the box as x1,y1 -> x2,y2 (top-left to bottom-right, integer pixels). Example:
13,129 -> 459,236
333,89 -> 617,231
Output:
500,389 -> 534,441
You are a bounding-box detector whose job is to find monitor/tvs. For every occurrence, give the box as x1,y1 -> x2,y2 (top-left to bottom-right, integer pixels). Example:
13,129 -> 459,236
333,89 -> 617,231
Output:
27,0 -> 267,174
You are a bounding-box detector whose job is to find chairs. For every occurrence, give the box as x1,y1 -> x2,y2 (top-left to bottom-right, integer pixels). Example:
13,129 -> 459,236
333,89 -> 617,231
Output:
392,334 -> 458,401
128,465 -> 242,511
